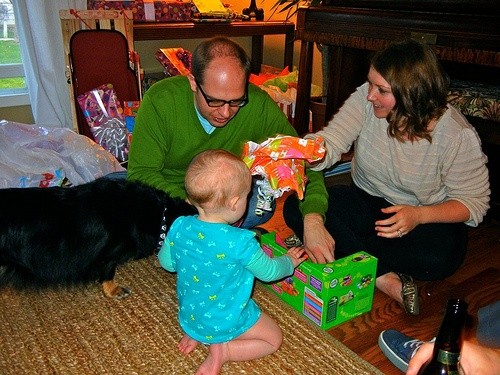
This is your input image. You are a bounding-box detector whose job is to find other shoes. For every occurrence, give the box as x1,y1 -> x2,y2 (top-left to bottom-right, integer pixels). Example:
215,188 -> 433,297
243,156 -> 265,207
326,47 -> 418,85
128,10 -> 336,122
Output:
393,270 -> 419,316
283,234 -> 303,248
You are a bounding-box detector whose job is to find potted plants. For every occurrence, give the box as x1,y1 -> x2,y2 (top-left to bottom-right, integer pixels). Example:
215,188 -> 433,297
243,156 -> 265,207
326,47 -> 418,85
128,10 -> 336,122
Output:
267,0 -> 326,136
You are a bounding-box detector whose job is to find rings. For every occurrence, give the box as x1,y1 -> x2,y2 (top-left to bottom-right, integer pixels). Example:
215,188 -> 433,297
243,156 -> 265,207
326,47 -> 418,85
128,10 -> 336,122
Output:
396,229 -> 403,238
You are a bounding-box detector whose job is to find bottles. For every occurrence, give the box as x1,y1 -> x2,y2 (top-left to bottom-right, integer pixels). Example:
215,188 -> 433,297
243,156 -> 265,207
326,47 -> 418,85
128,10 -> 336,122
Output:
415,299 -> 468,375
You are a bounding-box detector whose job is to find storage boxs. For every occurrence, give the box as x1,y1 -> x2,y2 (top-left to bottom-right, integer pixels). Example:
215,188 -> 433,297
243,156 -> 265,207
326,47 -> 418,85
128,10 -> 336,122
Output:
261,232 -> 378,331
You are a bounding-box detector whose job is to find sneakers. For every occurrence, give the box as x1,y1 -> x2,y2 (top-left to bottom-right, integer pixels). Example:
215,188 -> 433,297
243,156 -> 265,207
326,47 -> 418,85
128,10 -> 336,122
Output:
378,329 -> 428,372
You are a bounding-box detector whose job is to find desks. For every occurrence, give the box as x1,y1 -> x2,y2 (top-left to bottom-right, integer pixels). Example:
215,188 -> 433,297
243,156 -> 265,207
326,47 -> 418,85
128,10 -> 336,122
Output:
132,18 -> 295,73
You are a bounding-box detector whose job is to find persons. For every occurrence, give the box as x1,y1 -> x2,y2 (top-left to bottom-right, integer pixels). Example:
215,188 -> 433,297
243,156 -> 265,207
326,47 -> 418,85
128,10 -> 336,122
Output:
300,38 -> 490,316
104,35 -> 335,265
158,148 -> 306,375
377,301 -> 500,375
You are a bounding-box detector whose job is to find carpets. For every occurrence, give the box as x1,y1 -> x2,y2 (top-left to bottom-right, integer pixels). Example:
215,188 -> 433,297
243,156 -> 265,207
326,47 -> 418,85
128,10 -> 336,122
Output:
0,248 -> 385,375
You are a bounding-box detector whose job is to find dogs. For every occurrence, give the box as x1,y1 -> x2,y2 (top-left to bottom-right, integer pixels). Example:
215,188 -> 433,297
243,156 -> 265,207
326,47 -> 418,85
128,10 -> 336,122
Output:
0,176 -> 199,299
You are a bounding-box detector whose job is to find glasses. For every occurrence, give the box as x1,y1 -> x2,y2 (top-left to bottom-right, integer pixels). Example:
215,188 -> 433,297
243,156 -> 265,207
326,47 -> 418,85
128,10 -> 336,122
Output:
195,80 -> 248,107
